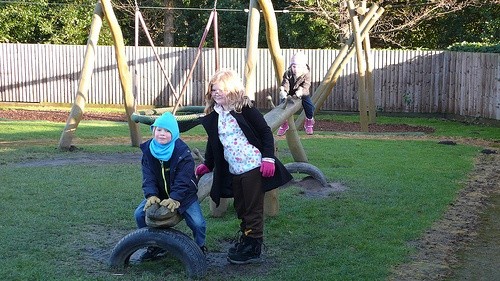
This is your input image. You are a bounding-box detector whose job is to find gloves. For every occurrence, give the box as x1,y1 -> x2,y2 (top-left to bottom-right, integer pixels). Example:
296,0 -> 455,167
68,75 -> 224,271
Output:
295,90 -> 302,97
260,161 -> 275,177
195,164 -> 210,175
280,90 -> 287,98
143,195 -> 160,211
159,198 -> 180,212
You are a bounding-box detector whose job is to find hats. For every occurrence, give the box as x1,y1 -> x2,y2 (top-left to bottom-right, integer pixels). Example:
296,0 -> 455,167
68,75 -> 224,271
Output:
288,52 -> 311,71
149,111 -> 180,161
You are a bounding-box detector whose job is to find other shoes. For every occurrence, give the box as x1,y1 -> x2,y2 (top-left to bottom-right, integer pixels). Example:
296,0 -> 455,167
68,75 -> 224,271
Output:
228,230 -> 248,252
226,234 -> 261,264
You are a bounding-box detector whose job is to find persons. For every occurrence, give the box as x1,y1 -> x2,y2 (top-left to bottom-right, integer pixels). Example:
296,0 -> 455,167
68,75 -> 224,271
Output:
195,70 -> 293,264
134,112 -> 211,261
277,53 -> 315,136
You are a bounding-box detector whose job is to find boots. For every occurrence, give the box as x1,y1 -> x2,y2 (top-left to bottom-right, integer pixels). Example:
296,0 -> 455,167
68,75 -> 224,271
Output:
277,120 -> 290,135
303,117 -> 314,134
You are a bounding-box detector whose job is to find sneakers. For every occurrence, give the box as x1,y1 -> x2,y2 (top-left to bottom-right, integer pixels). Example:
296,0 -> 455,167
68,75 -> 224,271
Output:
138,246 -> 168,261
200,246 -> 208,256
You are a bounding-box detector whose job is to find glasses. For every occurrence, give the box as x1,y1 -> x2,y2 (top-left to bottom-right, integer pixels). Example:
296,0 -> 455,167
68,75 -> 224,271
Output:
210,89 -> 225,96
